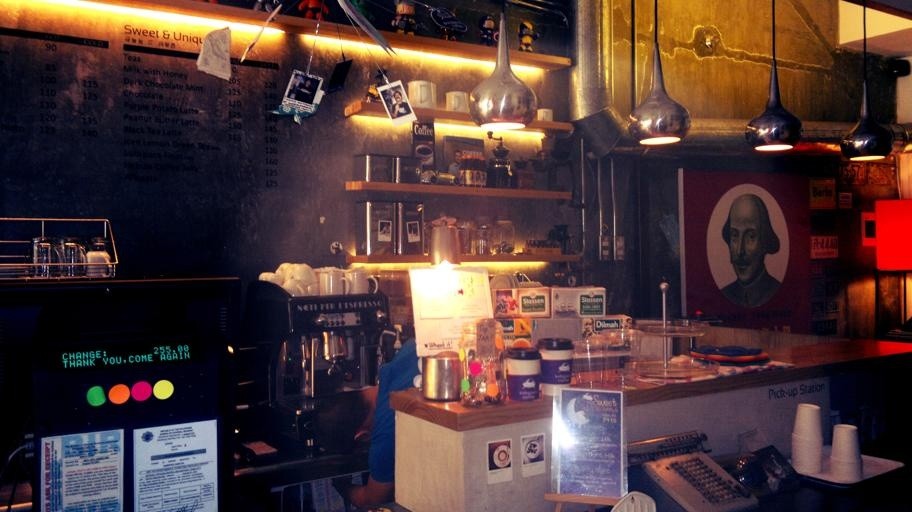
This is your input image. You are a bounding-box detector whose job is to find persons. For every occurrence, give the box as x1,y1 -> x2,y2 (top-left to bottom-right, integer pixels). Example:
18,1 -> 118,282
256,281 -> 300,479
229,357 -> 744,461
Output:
720,194 -> 782,306
295,77 -> 314,103
391,92 -> 410,117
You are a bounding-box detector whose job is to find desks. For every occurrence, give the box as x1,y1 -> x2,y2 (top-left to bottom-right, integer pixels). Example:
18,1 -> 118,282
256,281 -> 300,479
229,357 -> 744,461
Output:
594,447 -> 910,511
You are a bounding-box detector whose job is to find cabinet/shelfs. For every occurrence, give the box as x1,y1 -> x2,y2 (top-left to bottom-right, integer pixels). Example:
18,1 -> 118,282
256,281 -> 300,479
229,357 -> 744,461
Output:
341,99 -> 578,264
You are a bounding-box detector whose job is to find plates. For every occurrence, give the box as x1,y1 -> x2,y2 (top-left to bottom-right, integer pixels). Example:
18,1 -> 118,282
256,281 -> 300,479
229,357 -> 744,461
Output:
686,345 -> 772,366
415,158 -> 435,166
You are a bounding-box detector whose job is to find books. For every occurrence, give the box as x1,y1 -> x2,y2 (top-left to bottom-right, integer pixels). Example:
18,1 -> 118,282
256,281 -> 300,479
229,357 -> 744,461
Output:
558,390 -> 622,497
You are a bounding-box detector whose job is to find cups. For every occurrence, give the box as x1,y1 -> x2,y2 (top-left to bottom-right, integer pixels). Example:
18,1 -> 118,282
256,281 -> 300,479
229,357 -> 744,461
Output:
418,356 -> 463,402
536,108 -> 554,121
600,328 -> 644,389
407,80 -> 438,108
445,91 -> 469,113
415,144 -> 433,161
790,401 -> 827,481
507,343 -> 543,402
541,337 -> 577,403
258,261 -> 320,298
829,422 -> 868,485
567,335 -> 607,390
314,265 -> 379,296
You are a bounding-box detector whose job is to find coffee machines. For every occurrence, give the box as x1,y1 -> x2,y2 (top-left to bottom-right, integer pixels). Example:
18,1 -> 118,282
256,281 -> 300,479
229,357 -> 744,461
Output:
256,288 -> 393,454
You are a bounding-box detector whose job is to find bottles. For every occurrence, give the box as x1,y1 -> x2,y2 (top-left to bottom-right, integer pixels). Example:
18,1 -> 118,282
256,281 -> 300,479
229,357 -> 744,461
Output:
492,213 -> 516,256
54,236 -> 84,276
472,224 -> 491,256
458,153 -> 488,187
464,356 -> 502,408
31,236 -> 57,278
83,243 -> 111,279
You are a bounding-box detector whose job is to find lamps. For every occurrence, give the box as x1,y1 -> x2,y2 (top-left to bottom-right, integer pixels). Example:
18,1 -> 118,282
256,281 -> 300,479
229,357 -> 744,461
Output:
467,1 -> 539,133
837,3 -> 894,163
627,1 -> 691,148
744,1 -> 803,155
